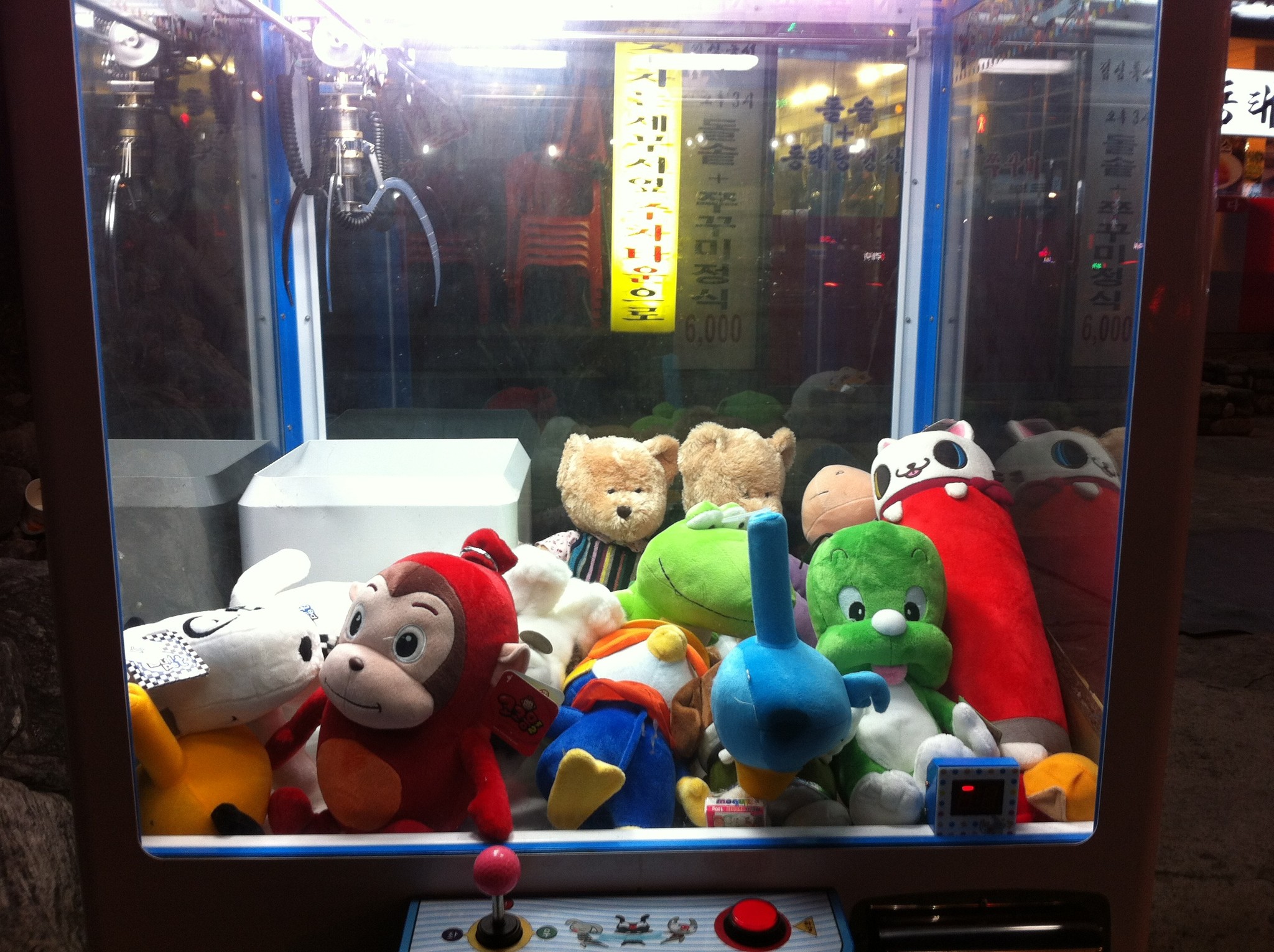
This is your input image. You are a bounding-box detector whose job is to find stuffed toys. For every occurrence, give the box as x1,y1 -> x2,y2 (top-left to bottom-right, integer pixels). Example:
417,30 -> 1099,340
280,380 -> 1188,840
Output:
677,417 -> 816,646
787,521 -> 1010,826
119,602 -> 325,736
492,366 -> 884,496
604,498 -> 798,649
230,542 -> 629,816
869,420 -> 1070,767
998,417 -> 1120,703
532,616 -> 723,830
127,679 -> 277,843
707,510 -> 893,804
535,429 -> 680,594
264,528 -> 517,846
800,462 -> 879,548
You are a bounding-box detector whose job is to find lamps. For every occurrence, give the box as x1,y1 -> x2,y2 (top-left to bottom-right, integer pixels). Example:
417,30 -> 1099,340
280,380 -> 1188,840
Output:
416,47 -> 760,75
